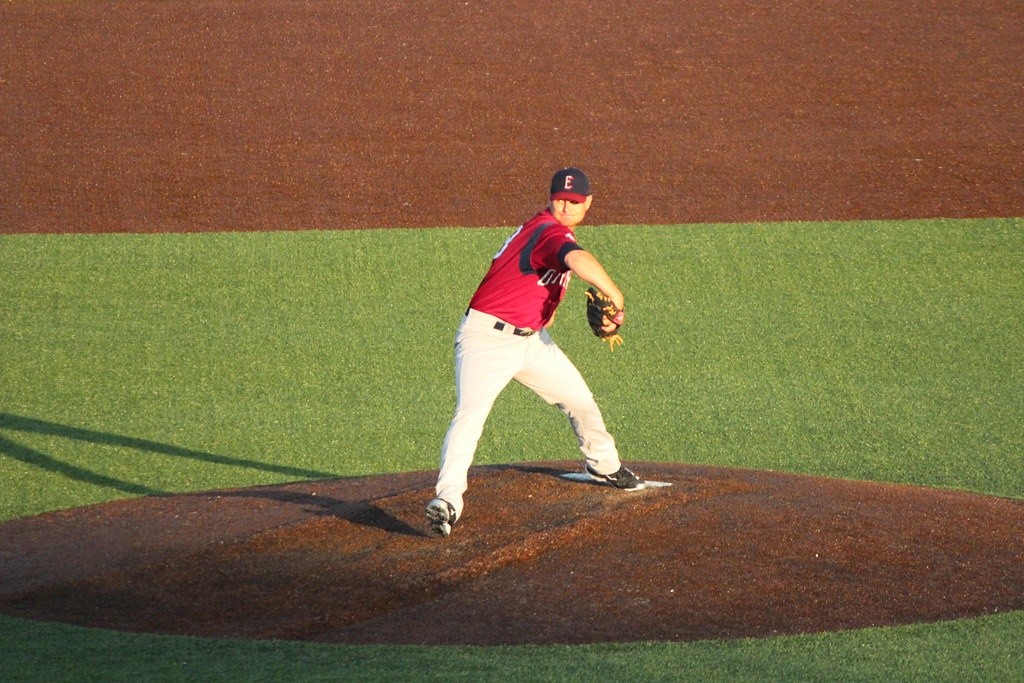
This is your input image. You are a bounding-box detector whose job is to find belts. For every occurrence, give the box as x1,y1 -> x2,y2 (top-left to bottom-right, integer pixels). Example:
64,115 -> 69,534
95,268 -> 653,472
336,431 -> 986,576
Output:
465,307 -> 535,337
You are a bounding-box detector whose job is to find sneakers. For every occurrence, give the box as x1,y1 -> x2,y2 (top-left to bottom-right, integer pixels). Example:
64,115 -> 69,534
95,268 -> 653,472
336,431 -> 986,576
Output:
426,498 -> 456,538
584,461 -> 646,491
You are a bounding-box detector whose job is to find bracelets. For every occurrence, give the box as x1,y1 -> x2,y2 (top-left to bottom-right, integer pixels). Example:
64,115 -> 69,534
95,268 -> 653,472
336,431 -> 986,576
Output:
611,310 -> 626,326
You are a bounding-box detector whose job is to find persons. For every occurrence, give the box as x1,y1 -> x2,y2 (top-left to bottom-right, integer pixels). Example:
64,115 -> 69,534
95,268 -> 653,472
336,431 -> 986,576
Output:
425,167 -> 646,537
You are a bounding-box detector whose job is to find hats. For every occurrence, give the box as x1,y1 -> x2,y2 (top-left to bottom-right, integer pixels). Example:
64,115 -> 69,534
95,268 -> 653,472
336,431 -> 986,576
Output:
549,167 -> 589,203
